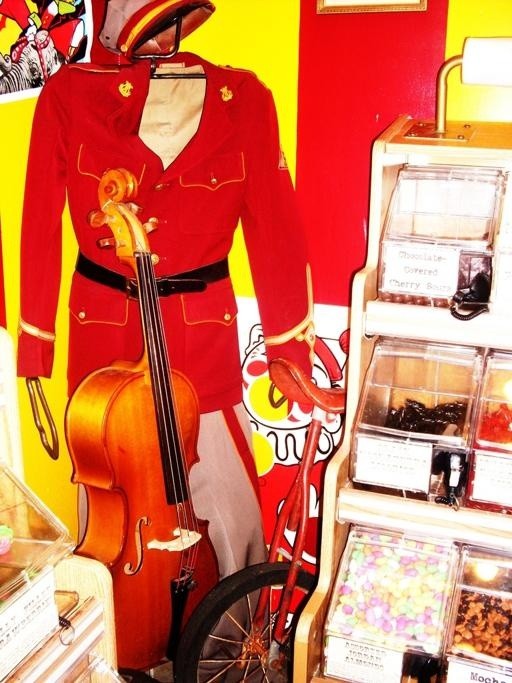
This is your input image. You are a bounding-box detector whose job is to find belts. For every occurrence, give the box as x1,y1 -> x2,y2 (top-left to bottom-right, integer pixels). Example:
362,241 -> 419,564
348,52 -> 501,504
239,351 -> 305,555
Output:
75,252 -> 229,299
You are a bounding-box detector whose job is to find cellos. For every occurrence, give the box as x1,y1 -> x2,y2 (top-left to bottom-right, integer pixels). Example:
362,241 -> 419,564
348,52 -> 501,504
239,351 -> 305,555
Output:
65,168 -> 219,673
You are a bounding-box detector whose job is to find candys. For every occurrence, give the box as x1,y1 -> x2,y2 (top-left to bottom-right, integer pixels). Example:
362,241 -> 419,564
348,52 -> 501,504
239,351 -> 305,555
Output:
334,535 -> 512,662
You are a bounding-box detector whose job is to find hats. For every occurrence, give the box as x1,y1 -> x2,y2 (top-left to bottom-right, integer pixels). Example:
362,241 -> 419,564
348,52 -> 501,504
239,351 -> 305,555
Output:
98,0 -> 216,56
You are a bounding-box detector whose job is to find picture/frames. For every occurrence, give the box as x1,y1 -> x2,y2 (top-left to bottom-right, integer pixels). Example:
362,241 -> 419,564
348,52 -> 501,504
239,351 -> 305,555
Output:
314,0 -> 428,14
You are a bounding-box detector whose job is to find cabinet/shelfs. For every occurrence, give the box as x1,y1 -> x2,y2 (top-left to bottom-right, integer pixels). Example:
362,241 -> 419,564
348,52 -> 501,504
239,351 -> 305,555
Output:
0,461 -> 115,683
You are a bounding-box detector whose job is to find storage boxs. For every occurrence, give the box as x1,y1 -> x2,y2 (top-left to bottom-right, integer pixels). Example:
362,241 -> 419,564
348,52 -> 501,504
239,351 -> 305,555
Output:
445,545 -> 512,682
348,336 -> 494,510
464,352 -> 512,516
324,523 -> 461,683
374,166 -> 507,308
